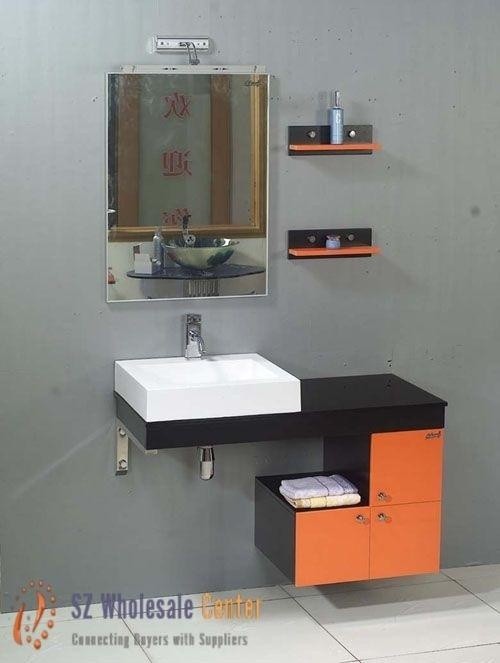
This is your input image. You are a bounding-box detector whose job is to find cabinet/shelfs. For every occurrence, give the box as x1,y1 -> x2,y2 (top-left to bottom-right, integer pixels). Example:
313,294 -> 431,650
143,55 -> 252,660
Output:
286,124 -> 381,260
114,373 -> 447,587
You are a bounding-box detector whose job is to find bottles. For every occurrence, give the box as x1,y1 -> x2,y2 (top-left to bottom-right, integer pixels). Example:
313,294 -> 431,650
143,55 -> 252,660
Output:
326,234 -> 341,250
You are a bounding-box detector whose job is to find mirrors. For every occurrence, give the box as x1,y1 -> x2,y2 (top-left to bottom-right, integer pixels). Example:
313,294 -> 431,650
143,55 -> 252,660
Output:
107,74 -> 264,241
105,71 -> 269,306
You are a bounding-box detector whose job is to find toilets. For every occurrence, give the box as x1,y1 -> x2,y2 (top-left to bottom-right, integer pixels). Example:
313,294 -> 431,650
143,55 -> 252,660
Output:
114,354 -> 302,424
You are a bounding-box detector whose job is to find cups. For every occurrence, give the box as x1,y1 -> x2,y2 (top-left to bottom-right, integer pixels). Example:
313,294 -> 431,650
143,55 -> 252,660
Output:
108,208 -> 116,227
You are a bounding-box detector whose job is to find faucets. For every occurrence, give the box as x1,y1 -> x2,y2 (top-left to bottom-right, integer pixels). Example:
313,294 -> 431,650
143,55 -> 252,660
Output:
182,213 -> 191,234
186,312 -> 207,359
